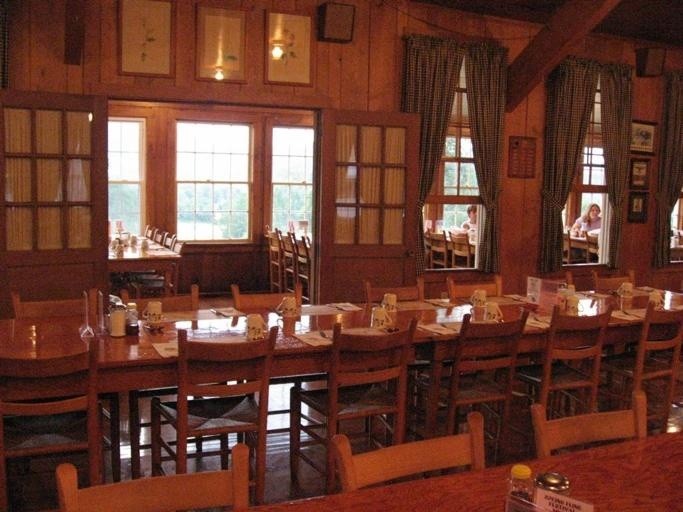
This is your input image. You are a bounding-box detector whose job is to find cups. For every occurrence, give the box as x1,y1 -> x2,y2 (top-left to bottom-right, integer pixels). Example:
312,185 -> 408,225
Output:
276,296 -> 298,318
245,313 -> 269,340
649,292 -> 665,311
438,225 -> 475,243
141,300 -> 163,325
370,307 -> 393,330
111,231 -> 150,256
469,289 -> 504,322
563,226 -> 569,233
616,283 -> 633,298
381,293 -> 397,312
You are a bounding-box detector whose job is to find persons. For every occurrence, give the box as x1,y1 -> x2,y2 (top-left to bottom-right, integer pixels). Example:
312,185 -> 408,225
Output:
459,205 -> 475,233
569,203 -> 601,235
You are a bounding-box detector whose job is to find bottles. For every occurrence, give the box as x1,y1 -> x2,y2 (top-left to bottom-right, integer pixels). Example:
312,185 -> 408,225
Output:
556,284 -> 579,316
507,464 -> 571,505
107,294 -> 139,337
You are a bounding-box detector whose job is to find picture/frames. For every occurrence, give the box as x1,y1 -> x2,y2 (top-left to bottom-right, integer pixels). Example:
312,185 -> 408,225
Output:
627,118 -> 659,224
116,0 -> 318,89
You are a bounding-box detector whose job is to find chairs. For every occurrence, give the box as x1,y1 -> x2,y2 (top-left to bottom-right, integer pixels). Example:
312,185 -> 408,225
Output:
563,229 -> 601,263
112,224 -> 186,293
424,229 -> 477,267
1,271 -> 682,512
265,226 -> 313,301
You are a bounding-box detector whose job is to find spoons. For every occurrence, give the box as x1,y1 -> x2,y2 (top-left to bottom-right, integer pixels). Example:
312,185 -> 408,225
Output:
622,310 -> 643,318
318,330 -> 333,339
